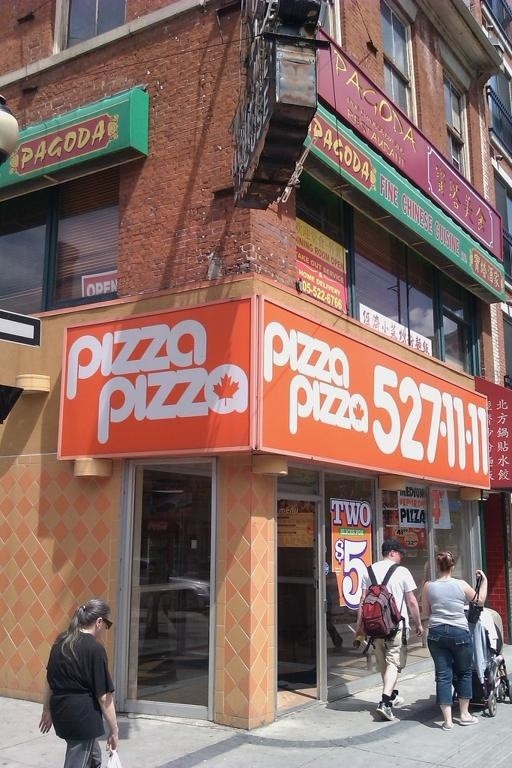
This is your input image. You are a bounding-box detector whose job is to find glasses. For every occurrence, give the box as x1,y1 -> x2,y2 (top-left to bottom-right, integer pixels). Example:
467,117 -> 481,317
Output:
101,618 -> 113,629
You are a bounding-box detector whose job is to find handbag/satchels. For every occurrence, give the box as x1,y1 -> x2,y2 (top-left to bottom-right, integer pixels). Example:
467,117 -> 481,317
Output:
468,601 -> 483,622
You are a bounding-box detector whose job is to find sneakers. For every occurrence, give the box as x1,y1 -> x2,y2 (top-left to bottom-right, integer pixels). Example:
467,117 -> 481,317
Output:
376,695 -> 404,720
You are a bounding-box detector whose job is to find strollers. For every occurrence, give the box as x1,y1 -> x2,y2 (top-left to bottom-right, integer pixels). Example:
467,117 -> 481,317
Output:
452,602 -> 512,718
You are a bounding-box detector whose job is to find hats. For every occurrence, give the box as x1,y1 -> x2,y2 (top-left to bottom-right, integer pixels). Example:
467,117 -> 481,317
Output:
383,539 -> 407,554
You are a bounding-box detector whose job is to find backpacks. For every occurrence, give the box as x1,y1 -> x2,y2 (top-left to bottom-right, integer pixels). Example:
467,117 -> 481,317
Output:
362,563 -> 401,639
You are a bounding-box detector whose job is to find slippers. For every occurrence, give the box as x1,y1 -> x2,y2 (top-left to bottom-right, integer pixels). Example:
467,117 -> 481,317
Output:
442,716 -> 478,730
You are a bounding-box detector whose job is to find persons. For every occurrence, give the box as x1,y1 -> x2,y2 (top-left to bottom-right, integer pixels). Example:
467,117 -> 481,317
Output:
421,551 -> 488,730
355,538 -> 424,721
38,600 -> 118,768
